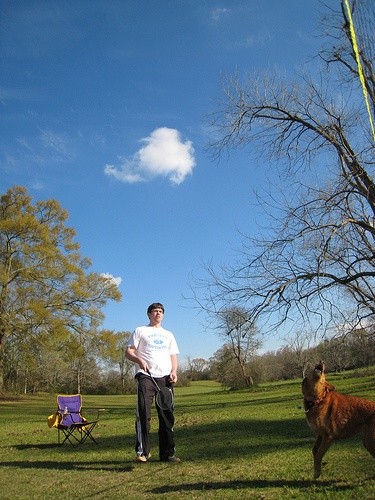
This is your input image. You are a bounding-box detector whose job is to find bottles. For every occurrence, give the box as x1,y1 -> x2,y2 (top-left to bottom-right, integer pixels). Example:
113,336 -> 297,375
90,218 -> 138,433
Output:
64,407 -> 68,413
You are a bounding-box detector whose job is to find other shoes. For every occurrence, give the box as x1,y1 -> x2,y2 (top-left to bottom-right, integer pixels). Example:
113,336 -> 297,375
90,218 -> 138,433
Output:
161,456 -> 180,462
136,453 -> 151,462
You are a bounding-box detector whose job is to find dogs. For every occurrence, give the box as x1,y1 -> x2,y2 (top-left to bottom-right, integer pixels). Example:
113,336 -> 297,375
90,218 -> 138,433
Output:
301,360 -> 375,479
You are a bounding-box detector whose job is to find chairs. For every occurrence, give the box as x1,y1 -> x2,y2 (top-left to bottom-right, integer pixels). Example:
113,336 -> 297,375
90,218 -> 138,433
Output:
56,394 -> 104,449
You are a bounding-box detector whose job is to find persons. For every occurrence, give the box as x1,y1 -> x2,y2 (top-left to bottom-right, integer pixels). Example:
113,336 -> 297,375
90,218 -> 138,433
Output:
123,303 -> 183,465
